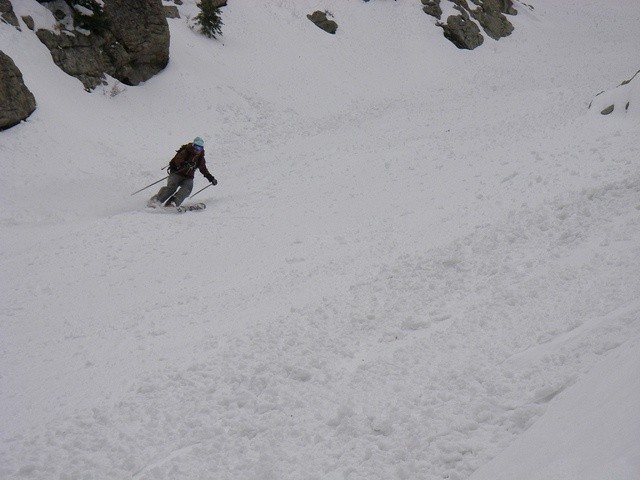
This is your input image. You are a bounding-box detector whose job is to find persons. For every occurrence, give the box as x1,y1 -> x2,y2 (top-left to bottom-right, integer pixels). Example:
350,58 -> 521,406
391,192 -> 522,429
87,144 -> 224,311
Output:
148,136 -> 217,210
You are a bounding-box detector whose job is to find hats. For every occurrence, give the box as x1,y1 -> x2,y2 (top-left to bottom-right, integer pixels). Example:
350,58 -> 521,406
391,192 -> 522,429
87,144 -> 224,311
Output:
193,137 -> 205,146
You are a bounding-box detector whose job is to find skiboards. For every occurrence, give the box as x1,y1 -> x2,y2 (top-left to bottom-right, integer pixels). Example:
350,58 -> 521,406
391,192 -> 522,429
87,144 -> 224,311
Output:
146,203 -> 207,214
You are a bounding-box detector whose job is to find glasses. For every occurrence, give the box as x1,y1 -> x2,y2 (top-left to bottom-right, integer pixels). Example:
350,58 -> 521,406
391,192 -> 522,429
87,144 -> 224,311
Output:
195,145 -> 203,150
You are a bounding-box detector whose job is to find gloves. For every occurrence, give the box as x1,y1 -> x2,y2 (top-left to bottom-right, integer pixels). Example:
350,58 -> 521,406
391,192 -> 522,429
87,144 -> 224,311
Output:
211,177 -> 217,186
